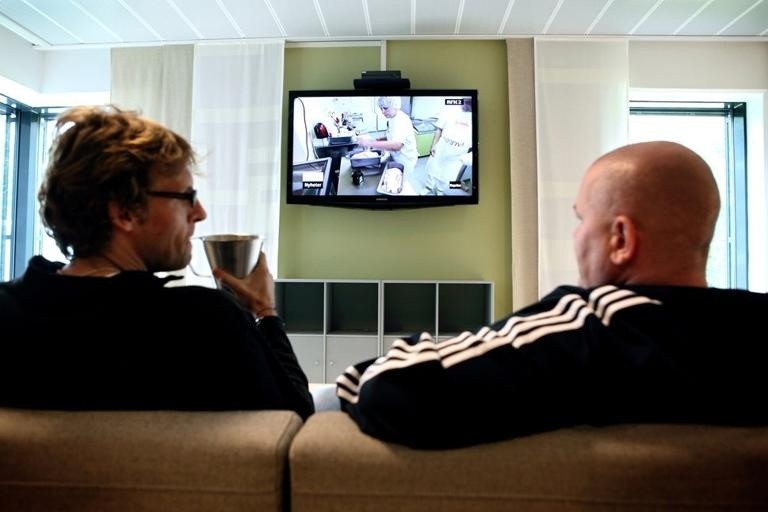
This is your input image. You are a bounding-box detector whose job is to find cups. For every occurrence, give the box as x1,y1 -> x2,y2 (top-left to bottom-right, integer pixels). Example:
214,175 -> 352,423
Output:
181,231 -> 264,295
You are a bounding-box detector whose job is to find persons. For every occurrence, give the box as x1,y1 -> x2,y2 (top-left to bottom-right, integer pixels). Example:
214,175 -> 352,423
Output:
2,106 -> 316,511
355,96 -> 418,196
422,98 -> 472,197
334,141 -> 768,451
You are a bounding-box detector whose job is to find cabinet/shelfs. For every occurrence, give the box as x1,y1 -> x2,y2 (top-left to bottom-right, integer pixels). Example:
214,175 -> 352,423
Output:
272,278 -> 380,384
380,279 -> 494,358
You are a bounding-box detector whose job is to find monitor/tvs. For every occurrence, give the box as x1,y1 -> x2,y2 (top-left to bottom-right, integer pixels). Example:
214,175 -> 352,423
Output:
287,90 -> 478,209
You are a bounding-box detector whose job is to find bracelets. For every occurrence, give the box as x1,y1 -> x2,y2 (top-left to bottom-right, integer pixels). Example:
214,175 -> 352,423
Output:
254,308 -> 278,323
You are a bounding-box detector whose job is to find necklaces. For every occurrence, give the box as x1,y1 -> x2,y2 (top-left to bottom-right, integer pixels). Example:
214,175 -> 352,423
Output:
81,267 -> 118,276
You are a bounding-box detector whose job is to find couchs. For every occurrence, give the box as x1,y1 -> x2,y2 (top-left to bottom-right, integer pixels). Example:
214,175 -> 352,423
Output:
0,405 -> 768,511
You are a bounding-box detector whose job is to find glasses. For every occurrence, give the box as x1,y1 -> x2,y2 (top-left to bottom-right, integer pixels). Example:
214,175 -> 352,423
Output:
149,188 -> 198,206
